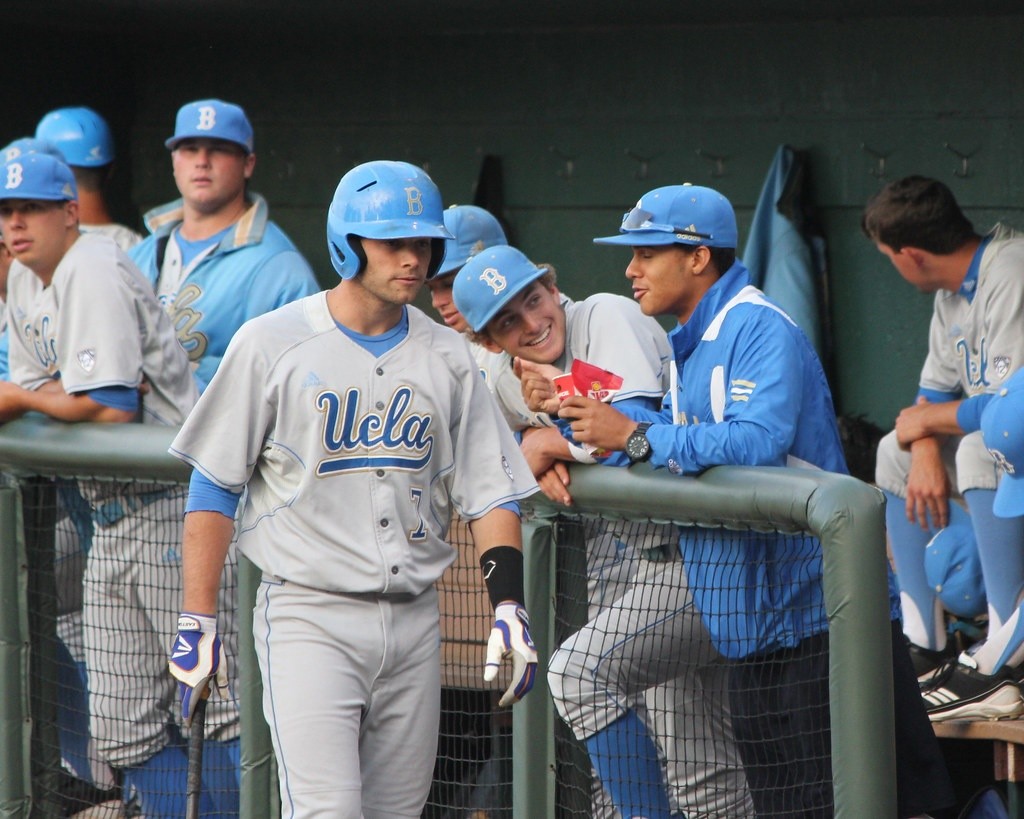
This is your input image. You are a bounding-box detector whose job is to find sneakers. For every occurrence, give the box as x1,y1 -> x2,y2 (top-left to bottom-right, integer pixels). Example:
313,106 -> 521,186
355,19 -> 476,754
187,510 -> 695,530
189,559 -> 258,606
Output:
914,647 -> 1024,721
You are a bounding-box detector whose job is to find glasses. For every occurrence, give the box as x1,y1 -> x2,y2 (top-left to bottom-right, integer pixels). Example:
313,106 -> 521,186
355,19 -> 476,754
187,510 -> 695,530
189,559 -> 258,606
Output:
618,207 -> 715,240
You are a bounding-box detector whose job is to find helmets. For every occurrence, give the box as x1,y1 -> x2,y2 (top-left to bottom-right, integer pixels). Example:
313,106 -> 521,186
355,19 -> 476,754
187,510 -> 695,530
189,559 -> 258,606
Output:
328,159 -> 456,280
34,108 -> 114,167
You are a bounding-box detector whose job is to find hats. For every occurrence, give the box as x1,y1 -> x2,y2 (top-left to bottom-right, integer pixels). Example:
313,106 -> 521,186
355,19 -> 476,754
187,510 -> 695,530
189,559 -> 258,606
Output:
1,139 -> 64,172
0,150 -> 77,204
432,204 -> 509,281
163,98 -> 253,156
452,245 -> 548,334
978,363 -> 1024,519
924,497 -> 989,620
593,183 -> 737,249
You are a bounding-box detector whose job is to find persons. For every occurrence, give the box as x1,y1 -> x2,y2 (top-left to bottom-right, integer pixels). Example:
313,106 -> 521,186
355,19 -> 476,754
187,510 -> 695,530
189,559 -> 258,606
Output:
427,202 -> 574,331
547,183 -> 960,819
452,245 -> 757,819
863,176 -> 1023,682
169,161 -> 541,819
919,367 -> 1023,722
0,100 -> 319,818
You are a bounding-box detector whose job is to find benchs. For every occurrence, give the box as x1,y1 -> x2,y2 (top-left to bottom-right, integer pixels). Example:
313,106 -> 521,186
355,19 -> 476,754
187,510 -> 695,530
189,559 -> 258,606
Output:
927,613 -> 1024,818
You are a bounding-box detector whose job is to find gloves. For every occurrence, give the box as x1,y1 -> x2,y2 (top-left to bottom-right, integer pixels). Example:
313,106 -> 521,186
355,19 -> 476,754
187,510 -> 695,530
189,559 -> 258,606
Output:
484,602 -> 542,707
167,612 -> 231,729
